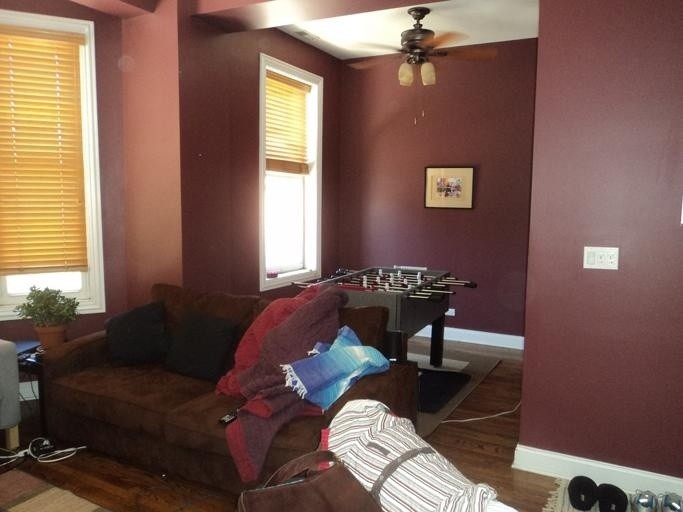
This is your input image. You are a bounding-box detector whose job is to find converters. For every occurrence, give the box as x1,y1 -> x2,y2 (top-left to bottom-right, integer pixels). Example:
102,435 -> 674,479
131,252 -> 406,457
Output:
36,439 -> 56,456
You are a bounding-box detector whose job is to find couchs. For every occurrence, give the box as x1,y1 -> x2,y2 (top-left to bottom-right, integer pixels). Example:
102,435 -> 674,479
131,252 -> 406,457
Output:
41,283 -> 419,497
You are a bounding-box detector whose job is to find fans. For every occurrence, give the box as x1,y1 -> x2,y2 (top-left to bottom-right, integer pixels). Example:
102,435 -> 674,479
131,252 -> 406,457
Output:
346,9 -> 496,86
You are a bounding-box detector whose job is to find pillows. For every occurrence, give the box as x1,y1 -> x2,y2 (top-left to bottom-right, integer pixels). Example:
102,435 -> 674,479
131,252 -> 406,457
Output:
99,297 -> 239,382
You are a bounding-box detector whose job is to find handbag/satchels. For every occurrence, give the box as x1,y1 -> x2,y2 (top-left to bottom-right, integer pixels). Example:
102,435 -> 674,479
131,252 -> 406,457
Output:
238,462 -> 383,511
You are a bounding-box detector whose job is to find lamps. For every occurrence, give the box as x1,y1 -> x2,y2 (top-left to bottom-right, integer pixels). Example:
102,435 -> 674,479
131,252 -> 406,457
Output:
397,54 -> 436,85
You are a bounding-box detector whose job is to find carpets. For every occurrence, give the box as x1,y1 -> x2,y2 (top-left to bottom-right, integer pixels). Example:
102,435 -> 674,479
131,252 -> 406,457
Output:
399,341 -> 502,441
0,469 -> 113,512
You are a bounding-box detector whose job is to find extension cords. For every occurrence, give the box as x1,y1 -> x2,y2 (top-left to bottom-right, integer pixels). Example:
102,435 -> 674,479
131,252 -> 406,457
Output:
17,448 -> 32,458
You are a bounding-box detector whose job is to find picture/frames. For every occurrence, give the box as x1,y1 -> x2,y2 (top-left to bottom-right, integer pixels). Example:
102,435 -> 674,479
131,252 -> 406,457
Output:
423,166 -> 472,209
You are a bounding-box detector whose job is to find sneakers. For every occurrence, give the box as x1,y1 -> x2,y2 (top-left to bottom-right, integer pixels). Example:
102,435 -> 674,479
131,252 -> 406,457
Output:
632,490 -> 655,511
657,491 -> 683,512
568,476 -> 599,510
598,483 -> 627,511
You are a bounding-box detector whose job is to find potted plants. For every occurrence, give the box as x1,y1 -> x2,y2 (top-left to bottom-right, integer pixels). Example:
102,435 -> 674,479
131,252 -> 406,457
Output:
13,285 -> 79,352
